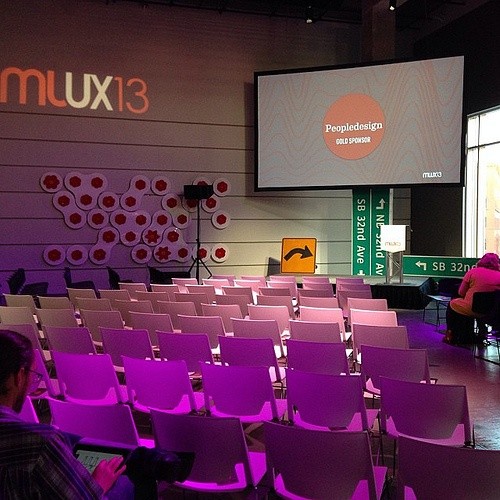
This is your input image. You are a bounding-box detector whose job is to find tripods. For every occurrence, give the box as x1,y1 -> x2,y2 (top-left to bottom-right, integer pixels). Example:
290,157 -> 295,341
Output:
188,199 -> 212,285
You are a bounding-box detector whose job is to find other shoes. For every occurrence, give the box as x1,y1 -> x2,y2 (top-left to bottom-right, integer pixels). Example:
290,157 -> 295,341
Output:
156,450 -> 196,483
443,335 -> 452,344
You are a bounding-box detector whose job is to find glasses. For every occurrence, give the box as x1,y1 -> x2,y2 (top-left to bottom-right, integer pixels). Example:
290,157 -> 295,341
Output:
29,369 -> 44,384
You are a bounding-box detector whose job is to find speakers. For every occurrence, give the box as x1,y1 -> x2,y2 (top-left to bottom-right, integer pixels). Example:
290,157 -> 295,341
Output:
184,185 -> 213,200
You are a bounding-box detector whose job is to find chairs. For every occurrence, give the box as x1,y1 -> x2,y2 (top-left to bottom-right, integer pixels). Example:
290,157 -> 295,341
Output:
0,267 -> 500,500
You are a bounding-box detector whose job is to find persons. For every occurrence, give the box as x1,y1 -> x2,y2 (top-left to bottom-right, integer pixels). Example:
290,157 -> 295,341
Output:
0,331 -> 196,500
444,253 -> 500,344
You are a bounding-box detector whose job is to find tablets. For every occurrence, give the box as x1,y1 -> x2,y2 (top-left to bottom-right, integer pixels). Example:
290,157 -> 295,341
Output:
72,442 -> 131,475
